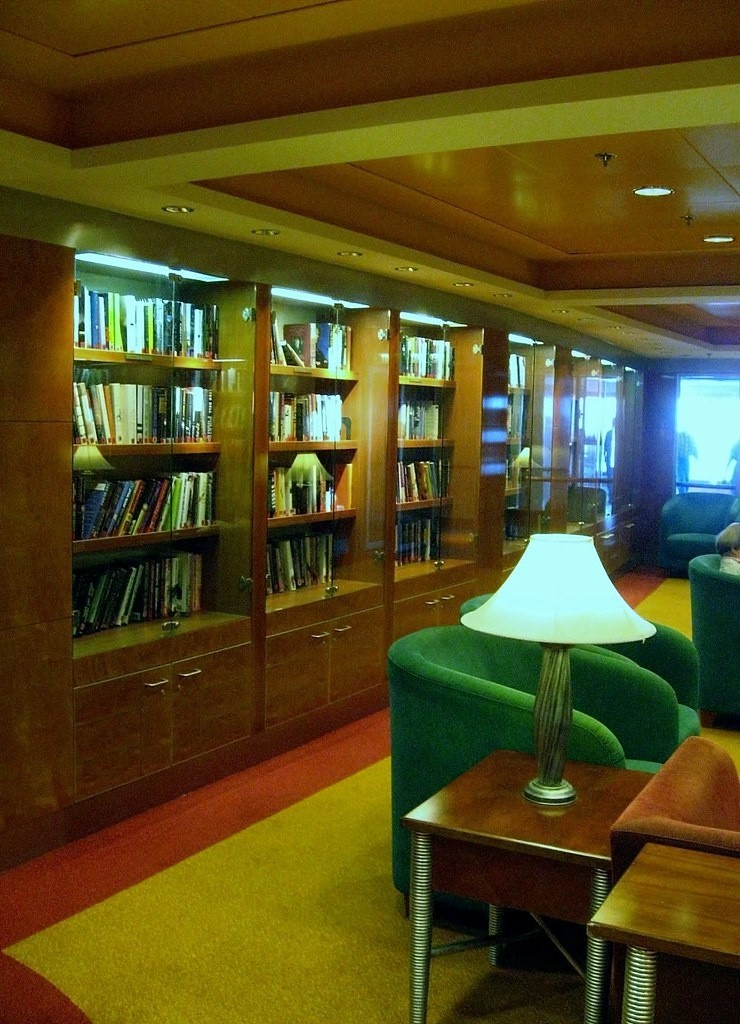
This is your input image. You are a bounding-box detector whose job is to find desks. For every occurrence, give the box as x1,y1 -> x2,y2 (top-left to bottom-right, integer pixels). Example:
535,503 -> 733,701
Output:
587,840 -> 740,1024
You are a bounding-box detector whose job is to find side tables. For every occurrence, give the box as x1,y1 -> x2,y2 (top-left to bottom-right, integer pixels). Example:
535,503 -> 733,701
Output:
401,746 -> 653,1024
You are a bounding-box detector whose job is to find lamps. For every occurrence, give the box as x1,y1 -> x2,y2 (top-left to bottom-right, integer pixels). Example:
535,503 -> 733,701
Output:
461,520 -> 657,809
71,435 -> 122,480
281,449 -> 338,492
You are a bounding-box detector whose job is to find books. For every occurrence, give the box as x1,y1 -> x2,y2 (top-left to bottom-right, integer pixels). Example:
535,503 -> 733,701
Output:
394,516 -> 439,568
267,532 -> 334,595
398,394 -> 444,439
74,471 -> 212,539
503,354 -> 526,542
396,459 -> 446,503
398,331 -> 455,379
269,391 -> 342,442
268,466 -> 337,518
72,279 -> 218,359
73,382 -> 214,446
72,551 -> 201,638
270,322 -> 352,370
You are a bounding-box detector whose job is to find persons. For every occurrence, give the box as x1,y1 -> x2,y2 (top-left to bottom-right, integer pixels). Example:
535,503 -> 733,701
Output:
727,441 -> 740,496
603,417 -> 616,507
677,431 -> 697,493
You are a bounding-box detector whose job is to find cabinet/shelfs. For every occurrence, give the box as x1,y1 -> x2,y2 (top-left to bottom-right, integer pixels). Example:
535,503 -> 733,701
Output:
0,236 -> 642,875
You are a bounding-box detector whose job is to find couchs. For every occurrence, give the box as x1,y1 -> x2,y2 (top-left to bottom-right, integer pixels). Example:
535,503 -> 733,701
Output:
458,587 -> 704,738
385,623 -> 677,915
689,549 -> 740,723
658,491 -> 740,577
600,722 -> 740,1024
